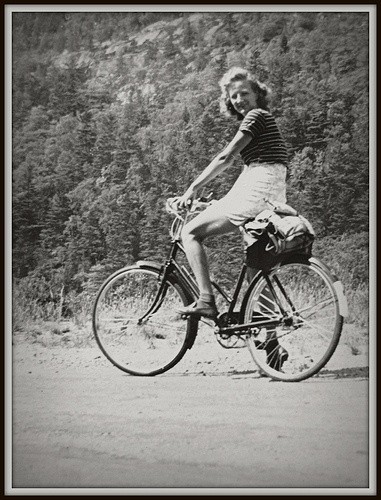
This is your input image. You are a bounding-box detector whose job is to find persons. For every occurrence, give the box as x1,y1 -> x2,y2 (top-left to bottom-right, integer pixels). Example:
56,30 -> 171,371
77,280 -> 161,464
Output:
167,65 -> 292,378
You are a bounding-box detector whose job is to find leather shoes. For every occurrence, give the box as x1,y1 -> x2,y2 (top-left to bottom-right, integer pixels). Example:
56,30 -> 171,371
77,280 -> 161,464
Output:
259,346 -> 288,377
175,299 -> 218,322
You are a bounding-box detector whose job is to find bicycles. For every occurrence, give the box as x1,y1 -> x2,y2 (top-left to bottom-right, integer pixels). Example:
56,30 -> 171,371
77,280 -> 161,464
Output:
91,191 -> 351,383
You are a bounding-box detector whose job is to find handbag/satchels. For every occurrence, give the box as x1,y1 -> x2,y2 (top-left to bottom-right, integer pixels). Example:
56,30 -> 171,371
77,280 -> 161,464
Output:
238,195 -> 316,272
